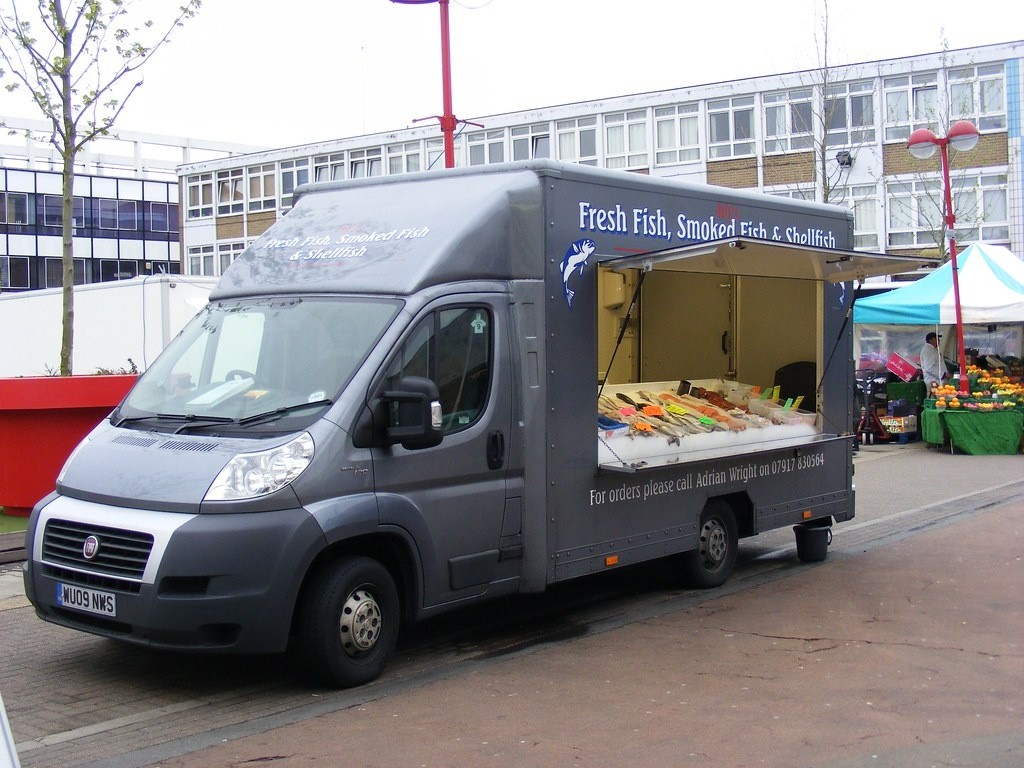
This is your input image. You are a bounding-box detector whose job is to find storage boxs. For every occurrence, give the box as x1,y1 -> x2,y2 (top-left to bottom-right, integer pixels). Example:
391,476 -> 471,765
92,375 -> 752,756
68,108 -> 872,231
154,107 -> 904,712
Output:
879,416 -> 892,425
889,416 -> 917,426
889,425 -> 918,433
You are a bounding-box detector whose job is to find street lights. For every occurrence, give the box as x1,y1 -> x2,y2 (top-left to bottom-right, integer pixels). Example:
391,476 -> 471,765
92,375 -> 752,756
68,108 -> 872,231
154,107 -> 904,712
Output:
906,119 -> 982,397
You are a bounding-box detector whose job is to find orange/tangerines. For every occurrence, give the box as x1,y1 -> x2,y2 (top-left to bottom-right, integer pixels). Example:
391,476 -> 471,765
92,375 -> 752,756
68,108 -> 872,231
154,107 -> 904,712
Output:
936,365 -> 1024,407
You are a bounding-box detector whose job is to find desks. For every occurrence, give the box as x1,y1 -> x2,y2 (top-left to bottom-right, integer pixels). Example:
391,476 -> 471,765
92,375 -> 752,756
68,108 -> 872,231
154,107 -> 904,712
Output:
920,352 -> 1024,455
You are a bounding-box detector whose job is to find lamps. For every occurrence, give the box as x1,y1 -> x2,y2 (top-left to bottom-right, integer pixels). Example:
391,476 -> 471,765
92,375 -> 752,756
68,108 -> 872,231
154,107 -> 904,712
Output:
836,151 -> 855,168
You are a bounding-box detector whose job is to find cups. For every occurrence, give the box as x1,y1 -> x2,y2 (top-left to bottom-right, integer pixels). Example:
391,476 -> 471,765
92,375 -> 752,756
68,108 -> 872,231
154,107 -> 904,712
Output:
677,380 -> 691,395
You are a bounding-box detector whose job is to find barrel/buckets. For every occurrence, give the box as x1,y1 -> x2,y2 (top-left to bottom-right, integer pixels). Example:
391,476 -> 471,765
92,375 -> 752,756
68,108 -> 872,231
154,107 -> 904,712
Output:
793,526 -> 833,562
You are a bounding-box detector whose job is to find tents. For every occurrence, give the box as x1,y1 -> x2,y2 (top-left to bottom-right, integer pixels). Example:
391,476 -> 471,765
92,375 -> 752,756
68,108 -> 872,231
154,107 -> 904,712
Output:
853,242 -> 1024,363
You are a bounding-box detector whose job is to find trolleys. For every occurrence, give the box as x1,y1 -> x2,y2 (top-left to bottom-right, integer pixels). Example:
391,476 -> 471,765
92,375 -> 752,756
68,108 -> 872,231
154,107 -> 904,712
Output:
855,368 -> 897,445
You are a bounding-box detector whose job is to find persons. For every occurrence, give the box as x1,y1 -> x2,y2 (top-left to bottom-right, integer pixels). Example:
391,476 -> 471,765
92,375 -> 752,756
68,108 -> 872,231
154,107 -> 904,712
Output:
921,332 -> 953,398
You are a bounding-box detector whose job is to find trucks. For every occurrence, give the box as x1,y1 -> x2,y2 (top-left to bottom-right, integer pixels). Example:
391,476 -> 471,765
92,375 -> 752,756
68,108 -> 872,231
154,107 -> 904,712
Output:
19,161 -> 946,690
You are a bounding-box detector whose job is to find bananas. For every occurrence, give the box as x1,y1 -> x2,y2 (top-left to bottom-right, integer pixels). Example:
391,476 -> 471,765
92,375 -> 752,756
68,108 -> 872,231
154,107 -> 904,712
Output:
968,369 -> 978,374
990,384 -> 999,389
1006,389 -> 1015,395
997,388 -> 1007,395
977,377 -> 988,383
1003,400 -> 1016,407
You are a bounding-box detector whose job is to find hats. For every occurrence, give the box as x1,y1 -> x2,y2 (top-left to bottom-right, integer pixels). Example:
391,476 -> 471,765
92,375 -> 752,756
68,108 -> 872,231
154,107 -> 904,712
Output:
926,333 -> 942,341
